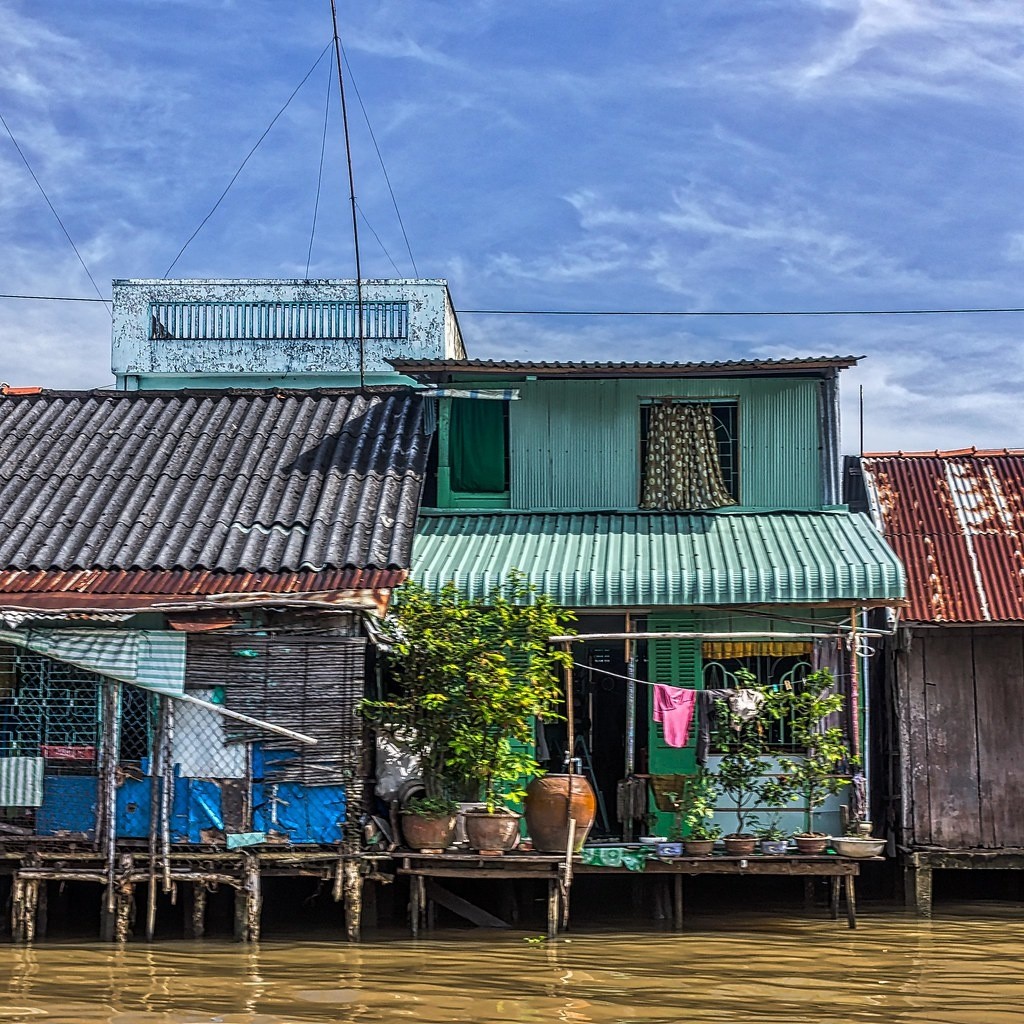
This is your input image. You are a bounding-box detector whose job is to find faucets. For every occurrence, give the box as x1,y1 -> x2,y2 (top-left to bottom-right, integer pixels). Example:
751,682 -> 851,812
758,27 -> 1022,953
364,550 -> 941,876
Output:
561,750 -> 574,764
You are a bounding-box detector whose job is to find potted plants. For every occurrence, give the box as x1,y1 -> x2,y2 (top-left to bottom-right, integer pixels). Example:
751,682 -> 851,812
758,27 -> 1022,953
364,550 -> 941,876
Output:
835,755 -> 887,859
663,763 -> 722,854
351,574 -> 488,850
778,665 -> 861,855
754,776 -> 792,853
417,562 -> 585,852
712,668 -> 798,854
